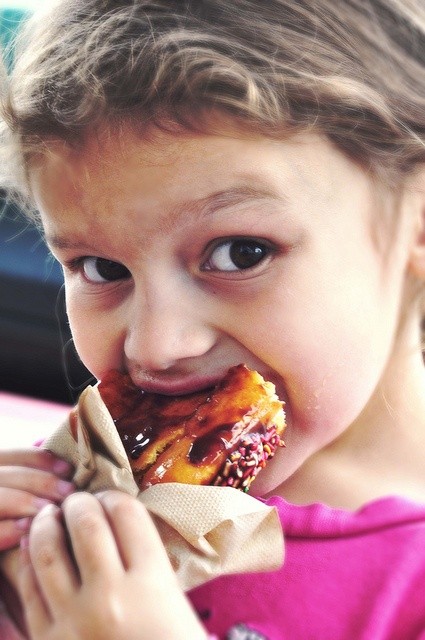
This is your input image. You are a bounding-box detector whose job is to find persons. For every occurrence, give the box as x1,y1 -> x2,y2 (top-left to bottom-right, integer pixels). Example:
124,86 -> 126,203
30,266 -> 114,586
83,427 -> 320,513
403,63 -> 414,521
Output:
0,1 -> 425,640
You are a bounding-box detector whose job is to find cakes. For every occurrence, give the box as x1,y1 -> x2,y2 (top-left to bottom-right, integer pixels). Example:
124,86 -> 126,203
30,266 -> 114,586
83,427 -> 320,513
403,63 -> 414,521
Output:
87,364 -> 288,498
